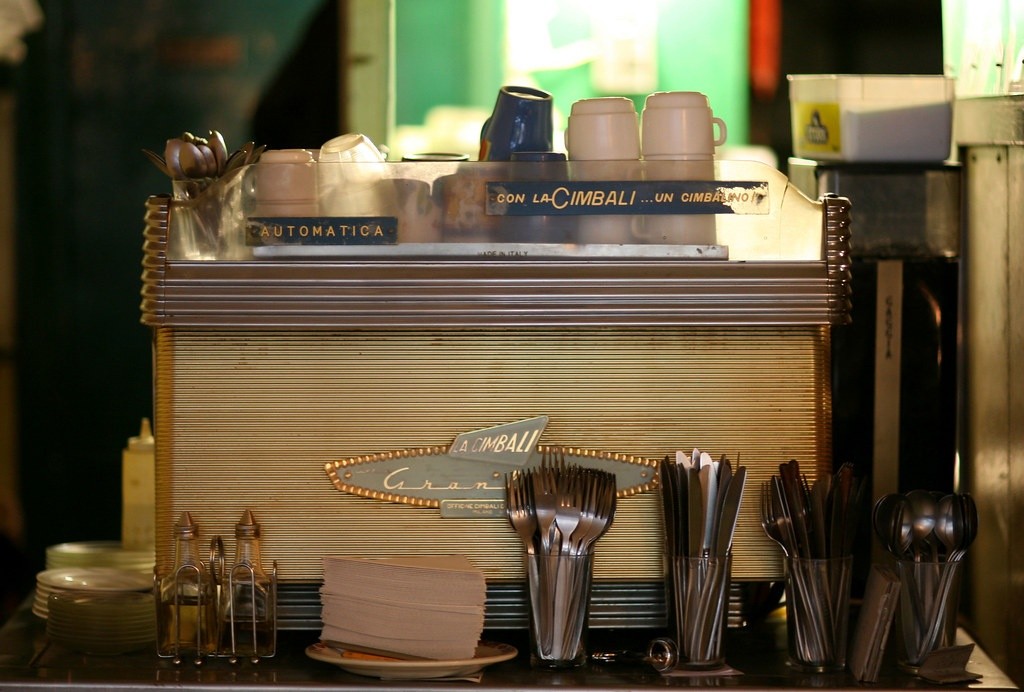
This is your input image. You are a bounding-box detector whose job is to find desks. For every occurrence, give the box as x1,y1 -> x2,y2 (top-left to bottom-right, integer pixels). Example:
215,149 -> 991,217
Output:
0,576 -> 1024,692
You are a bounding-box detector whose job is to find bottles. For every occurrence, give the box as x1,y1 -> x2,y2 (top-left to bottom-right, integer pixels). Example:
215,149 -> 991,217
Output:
120,417 -> 156,548
218,509 -> 273,657
160,511 -> 216,654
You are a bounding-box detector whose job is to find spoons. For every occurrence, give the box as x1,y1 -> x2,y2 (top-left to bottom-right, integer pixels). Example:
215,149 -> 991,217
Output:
140,127 -> 268,255
872,489 -> 981,665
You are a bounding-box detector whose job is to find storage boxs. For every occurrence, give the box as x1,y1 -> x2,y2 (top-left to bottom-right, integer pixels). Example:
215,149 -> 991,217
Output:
788,73 -> 957,162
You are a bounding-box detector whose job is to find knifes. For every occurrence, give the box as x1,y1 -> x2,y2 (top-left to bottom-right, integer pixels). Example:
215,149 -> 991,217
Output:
659,447 -> 750,660
758,458 -> 868,666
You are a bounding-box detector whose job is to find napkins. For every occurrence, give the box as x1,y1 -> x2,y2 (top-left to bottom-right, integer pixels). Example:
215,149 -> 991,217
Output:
318,557 -> 487,660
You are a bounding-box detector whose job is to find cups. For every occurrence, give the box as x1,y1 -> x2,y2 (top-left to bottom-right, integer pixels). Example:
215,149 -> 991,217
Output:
256,131 -> 471,246
892,556 -> 961,677
443,81 -> 728,247
524,554 -> 596,668
167,177 -> 223,262
660,552 -> 734,672
781,552 -> 853,674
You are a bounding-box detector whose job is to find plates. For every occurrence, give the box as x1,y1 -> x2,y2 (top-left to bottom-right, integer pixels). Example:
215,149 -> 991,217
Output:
304,640 -> 519,678
44,591 -> 156,660
31,565 -> 155,622
45,540 -> 157,572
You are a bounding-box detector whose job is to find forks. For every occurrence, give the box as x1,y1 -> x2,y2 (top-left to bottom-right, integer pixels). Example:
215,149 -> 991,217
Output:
502,446 -> 618,663
